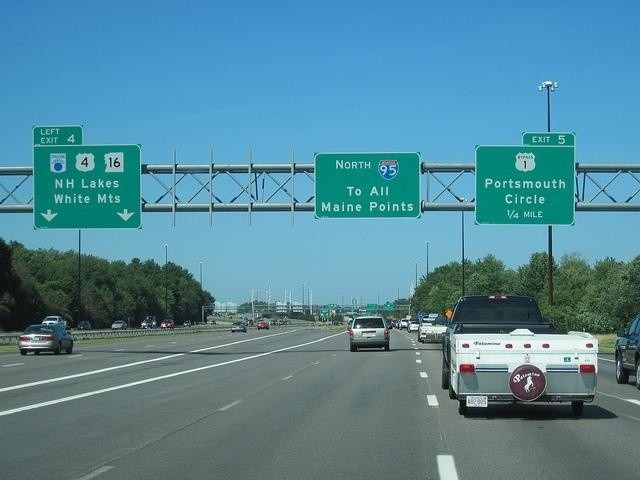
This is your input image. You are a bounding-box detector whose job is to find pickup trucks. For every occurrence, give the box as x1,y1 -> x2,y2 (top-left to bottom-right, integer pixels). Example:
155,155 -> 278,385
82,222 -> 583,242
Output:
441,296 -> 601,421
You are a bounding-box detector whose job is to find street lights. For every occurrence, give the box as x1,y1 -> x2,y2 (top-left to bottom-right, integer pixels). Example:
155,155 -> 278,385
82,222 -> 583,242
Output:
415,262 -> 418,289
302,281 -> 304,305
538,78 -> 561,307
396,285 -> 401,306
425,240 -> 431,278
164,244 -> 167,313
307,287 -> 309,305
199,261 -> 203,289
457,197 -> 471,297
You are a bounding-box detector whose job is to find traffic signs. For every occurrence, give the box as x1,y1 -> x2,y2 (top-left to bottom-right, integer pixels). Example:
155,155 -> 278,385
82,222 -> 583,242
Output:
314,150 -> 421,218
33,143 -> 143,231
33,124 -> 83,145
522,131 -> 577,147
474,144 -> 576,226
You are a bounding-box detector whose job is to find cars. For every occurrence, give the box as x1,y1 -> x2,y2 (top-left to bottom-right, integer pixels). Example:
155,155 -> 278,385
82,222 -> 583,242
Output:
614,314 -> 639,387
345,311 -> 449,351
210,313 -> 291,333
40,315 -> 192,336
18,324 -> 75,358
